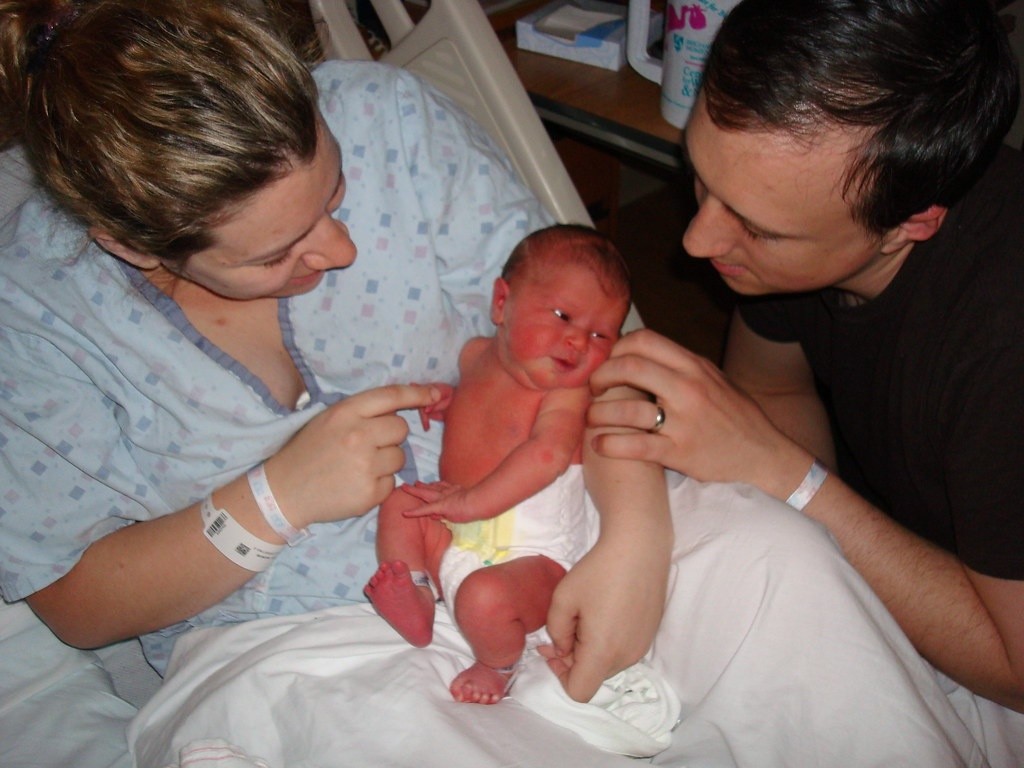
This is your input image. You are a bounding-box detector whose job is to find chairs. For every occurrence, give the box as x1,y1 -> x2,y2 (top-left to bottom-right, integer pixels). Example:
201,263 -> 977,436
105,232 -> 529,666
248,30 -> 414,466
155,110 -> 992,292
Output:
310,0 -> 645,341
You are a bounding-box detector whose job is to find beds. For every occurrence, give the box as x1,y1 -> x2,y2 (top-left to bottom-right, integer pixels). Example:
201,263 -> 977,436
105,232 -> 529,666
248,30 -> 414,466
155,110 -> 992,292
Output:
0,143 -> 1024,768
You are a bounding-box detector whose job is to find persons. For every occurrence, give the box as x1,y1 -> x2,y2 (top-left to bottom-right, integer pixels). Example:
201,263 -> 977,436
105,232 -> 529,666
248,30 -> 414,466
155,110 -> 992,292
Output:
365,224 -> 631,703
0,0 -> 676,766
584,0 -> 1024,713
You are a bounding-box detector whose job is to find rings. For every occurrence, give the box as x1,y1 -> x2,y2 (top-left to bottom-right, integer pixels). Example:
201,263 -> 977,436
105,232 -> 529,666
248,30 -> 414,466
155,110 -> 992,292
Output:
648,405 -> 663,431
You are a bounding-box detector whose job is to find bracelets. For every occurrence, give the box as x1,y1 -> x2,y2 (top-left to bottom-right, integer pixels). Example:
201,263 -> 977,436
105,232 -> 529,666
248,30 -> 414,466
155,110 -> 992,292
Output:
199,493 -> 280,573
787,459 -> 830,512
248,463 -> 304,545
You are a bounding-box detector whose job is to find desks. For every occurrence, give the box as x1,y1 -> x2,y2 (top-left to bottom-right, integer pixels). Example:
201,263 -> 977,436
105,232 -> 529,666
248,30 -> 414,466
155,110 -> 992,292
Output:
404,0 -> 688,170
